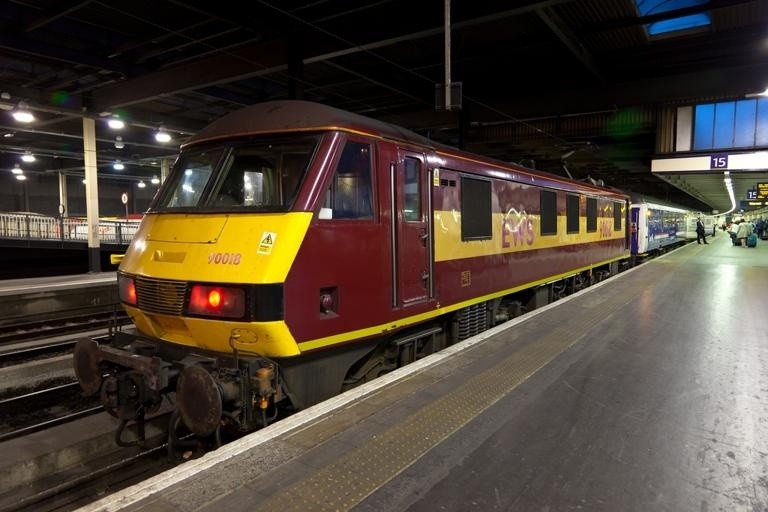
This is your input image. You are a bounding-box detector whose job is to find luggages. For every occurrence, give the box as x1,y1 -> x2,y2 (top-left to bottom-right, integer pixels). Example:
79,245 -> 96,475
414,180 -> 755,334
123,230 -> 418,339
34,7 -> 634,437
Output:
747,234 -> 756,248
735,239 -> 742,246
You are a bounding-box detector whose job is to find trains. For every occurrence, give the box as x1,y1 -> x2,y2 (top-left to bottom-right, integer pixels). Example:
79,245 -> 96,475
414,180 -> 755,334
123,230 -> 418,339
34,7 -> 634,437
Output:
73,99 -> 718,466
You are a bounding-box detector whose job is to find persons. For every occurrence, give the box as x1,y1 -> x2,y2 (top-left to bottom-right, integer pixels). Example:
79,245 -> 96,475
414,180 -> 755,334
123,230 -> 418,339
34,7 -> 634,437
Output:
695,217 -> 708,244
721,217 -> 768,248
